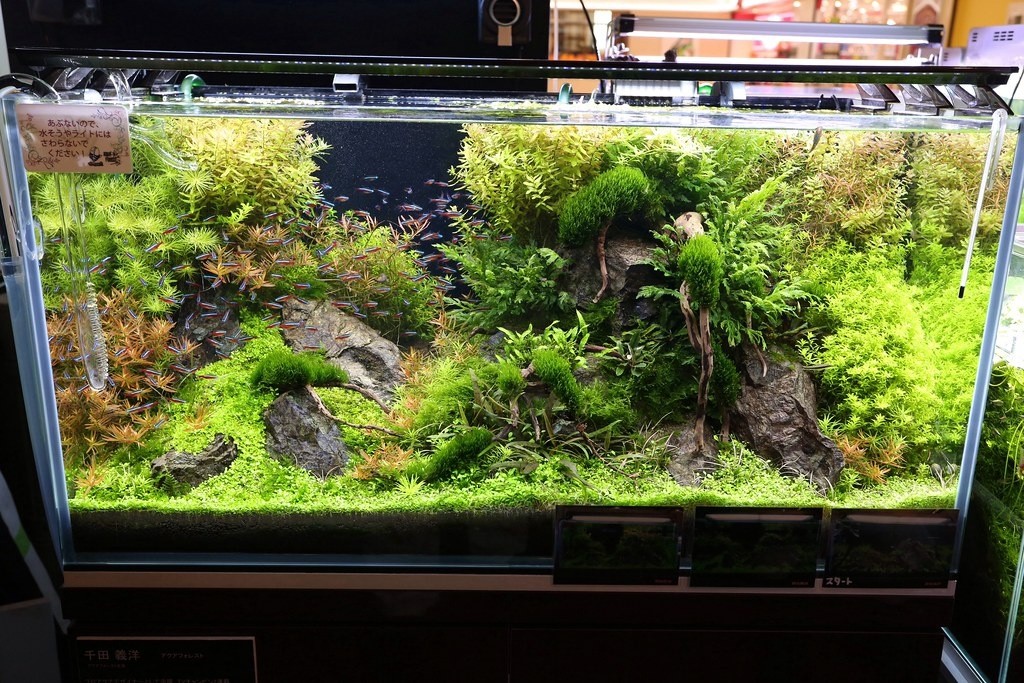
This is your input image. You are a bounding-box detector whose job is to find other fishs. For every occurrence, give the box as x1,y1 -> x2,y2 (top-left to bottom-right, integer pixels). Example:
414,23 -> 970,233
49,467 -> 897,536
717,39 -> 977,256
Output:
47,174 -> 512,433
